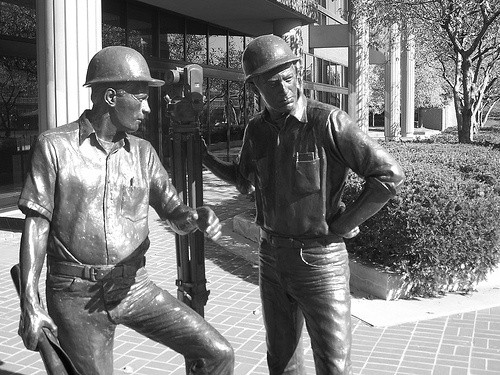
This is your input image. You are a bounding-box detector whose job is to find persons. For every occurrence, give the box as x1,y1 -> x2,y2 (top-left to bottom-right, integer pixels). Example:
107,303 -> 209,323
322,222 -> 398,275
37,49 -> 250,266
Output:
194,31 -> 403,374
17,45 -> 240,375
